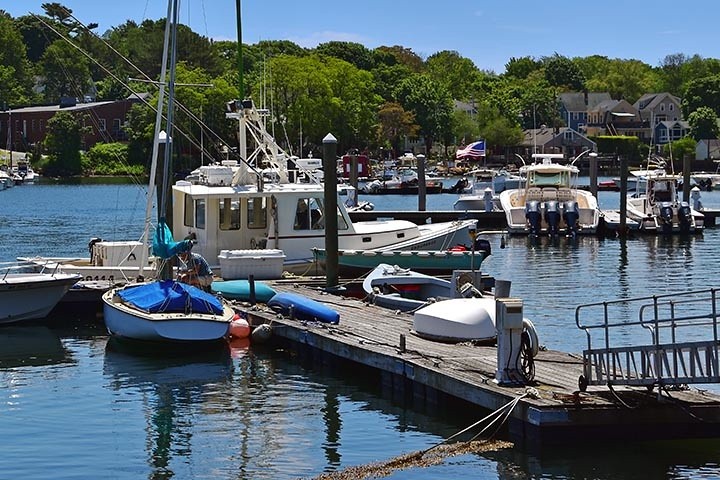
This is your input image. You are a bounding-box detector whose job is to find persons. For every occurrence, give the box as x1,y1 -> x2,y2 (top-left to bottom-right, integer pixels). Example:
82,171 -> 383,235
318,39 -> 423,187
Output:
293,198 -> 321,231
178,250 -> 214,290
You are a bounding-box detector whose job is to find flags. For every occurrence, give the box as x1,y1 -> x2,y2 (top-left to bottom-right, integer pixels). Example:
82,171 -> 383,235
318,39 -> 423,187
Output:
456,141 -> 486,159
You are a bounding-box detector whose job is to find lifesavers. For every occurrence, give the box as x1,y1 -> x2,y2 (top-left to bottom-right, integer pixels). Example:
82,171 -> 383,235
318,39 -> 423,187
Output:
522,316 -> 539,359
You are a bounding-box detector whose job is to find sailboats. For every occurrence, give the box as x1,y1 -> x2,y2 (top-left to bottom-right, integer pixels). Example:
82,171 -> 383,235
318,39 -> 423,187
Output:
102,0 -> 234,344
612,111 -> 685,194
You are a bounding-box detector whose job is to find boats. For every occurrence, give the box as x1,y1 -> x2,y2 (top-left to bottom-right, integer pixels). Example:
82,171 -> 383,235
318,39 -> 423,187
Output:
677,168 -> 720,221
300,146 -> 522,195
0,320 -> 78,370
603,120 -> 706,234
362,261 -> 488,316
309,244 -> 486,278
499,153 -> 601,241
172,60 -> 478,273
0,258 -> 84,326
14,240 -> 189,282
0,107 -> 40,190
